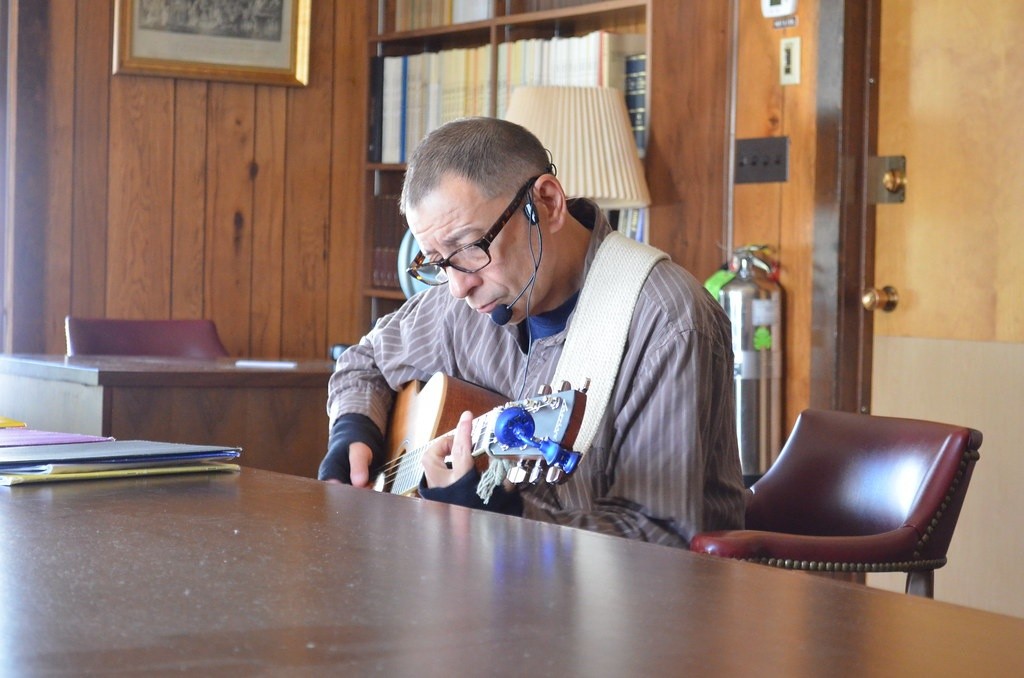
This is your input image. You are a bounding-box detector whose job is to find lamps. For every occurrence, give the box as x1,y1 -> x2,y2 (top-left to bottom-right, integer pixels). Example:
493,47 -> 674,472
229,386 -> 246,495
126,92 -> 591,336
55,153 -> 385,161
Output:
505,85 -> 652,210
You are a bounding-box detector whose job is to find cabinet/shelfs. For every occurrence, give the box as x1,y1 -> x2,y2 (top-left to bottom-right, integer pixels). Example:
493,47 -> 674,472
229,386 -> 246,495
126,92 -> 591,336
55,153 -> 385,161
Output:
360,0 -> 736,340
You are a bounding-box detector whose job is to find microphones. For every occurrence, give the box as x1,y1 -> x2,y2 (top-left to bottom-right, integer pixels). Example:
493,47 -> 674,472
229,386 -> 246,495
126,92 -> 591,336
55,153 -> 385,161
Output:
489,203 -> 543,325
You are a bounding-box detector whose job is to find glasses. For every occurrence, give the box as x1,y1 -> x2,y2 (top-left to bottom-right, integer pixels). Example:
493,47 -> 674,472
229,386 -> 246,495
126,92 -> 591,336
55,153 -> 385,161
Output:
408,175 -> 547,286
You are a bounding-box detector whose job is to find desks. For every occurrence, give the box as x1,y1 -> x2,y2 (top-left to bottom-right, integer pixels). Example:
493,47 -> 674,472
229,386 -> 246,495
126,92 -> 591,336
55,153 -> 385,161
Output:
1,352 -> 338,480
0,464 -> 1024,678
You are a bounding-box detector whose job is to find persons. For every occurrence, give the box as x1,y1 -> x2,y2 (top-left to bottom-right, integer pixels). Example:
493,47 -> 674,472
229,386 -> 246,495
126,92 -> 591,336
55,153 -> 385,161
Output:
316,115 -> 748,552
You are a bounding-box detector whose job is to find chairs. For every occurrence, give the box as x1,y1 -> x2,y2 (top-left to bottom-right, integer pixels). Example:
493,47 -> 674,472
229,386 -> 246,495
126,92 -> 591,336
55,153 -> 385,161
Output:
696,408 -> 983,600
65,317 -> 231,358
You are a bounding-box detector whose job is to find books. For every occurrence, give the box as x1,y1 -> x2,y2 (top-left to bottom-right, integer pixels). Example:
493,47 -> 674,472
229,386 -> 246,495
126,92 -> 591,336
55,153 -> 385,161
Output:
369,1 -> 647,294
0,440 -> 243,469
0,463 -> 235,476
1,474 -> 241,485
0,416 -> 27,429
1,429 -> 116,447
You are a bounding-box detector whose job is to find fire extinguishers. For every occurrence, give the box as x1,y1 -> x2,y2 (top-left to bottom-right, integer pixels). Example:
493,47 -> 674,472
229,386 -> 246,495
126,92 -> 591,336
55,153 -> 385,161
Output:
719,242 -> 785,480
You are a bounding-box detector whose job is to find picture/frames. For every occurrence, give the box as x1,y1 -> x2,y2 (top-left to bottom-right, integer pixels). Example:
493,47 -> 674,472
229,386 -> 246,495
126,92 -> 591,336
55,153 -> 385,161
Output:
112,0 -> 314,88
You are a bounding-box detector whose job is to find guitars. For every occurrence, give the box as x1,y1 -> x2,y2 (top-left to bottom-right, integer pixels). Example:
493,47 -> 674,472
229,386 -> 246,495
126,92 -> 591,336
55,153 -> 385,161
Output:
346,370 -> 591,497
